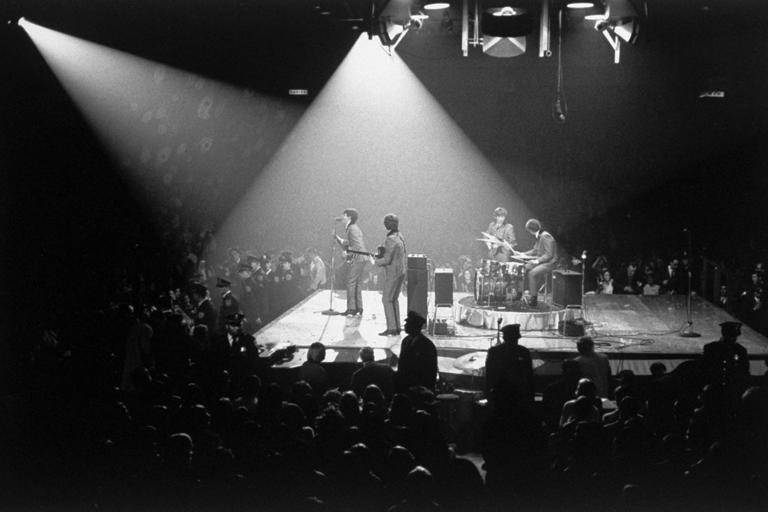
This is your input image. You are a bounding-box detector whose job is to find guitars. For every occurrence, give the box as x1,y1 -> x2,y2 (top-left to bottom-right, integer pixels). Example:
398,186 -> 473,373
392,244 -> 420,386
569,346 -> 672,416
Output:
346,247 -> 385,258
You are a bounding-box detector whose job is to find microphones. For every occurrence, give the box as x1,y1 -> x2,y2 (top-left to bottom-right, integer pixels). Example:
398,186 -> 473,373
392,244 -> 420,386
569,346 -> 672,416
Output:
333,216 -> 343,224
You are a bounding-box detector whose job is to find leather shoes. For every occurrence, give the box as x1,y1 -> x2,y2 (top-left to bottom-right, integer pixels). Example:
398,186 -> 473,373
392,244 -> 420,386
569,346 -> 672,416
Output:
341,308 -> 365,318
378,327 -> 402,337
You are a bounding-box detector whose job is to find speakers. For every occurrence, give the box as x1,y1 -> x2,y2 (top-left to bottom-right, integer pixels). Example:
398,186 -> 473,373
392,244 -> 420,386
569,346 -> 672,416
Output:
406,268 -> 428,329
552,269 -> 583,308
434,267 -> 454,305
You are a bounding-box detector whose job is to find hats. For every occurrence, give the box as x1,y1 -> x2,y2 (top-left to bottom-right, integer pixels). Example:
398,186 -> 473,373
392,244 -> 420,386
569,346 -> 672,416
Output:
718,321 -> 743,338
498,323 -> 523,340
215,277 -> 232,288
225,312 -> 246,324
191,283 -> 208,294
403,310 -> 427,324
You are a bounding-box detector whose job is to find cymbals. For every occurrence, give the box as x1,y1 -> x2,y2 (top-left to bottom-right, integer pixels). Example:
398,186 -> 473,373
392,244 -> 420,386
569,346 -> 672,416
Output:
478,231 -> 501,243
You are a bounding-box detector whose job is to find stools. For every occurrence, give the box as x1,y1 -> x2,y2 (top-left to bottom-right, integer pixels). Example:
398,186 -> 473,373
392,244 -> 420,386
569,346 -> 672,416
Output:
537,267 -> 554,304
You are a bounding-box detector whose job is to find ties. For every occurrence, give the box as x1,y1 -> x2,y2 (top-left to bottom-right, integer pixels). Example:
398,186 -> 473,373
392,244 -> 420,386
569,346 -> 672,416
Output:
238,254 -> 292,273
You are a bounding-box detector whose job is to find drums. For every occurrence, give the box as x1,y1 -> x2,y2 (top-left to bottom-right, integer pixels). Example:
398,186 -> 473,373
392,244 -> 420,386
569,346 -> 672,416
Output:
473,270 -> 505,303
503,262 -> 525,277
480,259 -> 499,273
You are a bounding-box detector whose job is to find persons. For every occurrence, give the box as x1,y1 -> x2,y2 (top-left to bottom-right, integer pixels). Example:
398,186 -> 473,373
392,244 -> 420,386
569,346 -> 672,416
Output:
38,205 -> 768,511
